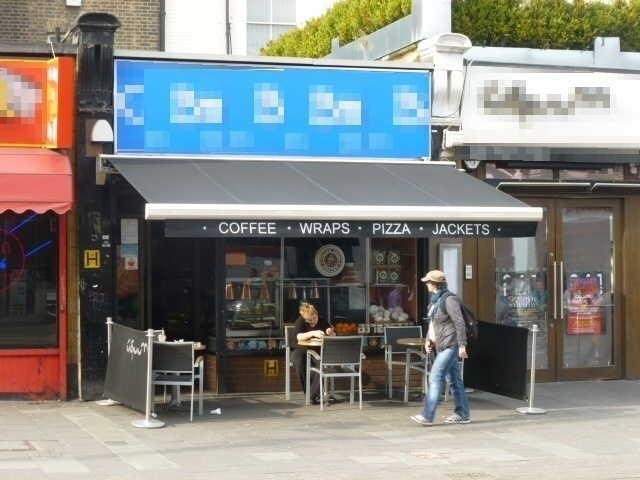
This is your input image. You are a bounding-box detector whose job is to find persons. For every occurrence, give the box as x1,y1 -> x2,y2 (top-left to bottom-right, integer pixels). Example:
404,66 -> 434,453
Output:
409,268 -> 472,426
290,301 -> 338,405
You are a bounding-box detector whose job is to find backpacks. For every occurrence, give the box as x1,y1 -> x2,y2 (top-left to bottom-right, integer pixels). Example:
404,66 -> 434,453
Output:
440,292 -> 478,337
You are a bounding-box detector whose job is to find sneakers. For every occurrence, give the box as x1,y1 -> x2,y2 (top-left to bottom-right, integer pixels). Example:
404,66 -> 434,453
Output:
410,414 -> 433,426
444,413 -> 471,423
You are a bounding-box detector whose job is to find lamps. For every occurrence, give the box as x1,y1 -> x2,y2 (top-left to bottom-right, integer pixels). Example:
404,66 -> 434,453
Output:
85,118 -> 114,157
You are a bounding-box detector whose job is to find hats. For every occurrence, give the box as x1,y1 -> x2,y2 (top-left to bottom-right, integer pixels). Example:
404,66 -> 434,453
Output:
420,270 -> 446,282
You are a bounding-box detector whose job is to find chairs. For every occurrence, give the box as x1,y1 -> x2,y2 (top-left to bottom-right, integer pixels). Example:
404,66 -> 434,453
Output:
144,330 -> 167,404
283,325 -> 464,412
152,342 -> 204,422
